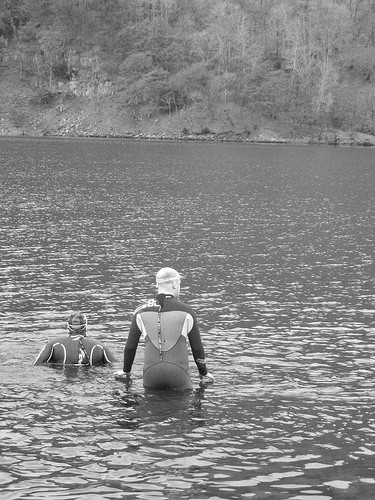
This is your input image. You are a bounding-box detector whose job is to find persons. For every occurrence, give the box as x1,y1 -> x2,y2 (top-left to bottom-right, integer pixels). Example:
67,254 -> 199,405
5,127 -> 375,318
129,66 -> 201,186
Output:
32,312 -> 118,367
111,267 -> 217,391
